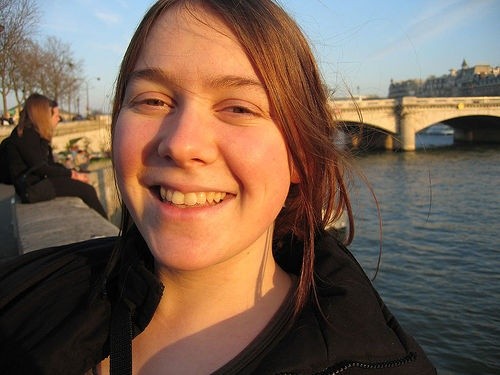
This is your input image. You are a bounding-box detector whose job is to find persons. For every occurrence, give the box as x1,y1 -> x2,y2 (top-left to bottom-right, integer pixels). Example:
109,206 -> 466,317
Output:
0,93 -> 109,222
0,0 -> 437,375
